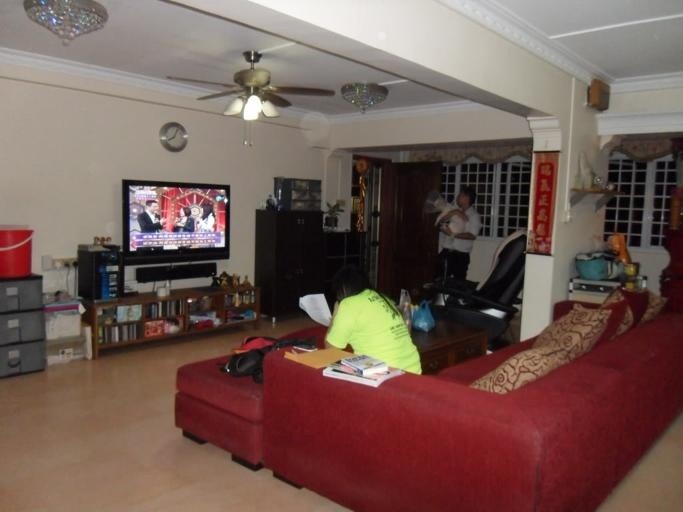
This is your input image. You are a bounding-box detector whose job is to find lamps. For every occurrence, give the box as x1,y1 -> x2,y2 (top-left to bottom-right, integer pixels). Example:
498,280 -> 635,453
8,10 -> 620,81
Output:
228,92 -> 277,130
338,83 -> 392,116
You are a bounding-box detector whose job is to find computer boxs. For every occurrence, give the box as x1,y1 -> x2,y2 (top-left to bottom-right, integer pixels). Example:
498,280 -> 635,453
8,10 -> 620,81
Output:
77,250 -> 124,300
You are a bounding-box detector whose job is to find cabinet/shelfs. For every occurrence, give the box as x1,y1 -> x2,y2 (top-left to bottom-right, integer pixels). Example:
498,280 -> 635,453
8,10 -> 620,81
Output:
90,281 -> 266,357
569,188 -> 625,211
325,232 -> 368,315
570,274 -> 648,300
255,206 -> 323,323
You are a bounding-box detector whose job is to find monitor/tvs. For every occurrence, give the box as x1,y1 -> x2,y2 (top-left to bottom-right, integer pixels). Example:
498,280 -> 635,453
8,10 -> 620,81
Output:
122,179 -> 230,266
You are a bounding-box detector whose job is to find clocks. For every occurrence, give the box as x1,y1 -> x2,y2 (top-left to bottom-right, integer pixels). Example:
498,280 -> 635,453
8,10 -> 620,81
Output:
157,120 -> 188,153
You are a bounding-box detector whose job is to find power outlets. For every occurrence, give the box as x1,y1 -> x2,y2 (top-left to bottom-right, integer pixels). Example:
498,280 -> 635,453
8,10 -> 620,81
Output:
18,2 -> 110,47
43,257 -> 78,272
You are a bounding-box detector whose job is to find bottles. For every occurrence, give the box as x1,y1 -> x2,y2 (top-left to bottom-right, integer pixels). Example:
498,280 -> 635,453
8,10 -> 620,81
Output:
243,291 -> 255,305
641,275 -> 647,288
266,186 -> 285,213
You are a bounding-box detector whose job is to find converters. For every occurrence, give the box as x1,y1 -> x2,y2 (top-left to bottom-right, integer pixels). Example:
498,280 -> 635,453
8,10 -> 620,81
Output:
54,260 -> 62,268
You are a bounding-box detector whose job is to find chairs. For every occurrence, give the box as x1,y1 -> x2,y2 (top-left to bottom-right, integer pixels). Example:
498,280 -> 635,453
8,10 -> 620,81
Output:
421,229 -> 527,322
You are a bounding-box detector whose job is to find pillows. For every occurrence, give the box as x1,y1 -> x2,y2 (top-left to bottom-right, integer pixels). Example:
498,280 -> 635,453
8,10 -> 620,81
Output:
473,345 -> 570,391
529,302 -> 607,353
601,286 -> 668,336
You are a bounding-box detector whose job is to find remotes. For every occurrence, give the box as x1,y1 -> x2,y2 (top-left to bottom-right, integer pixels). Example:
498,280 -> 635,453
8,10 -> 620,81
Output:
292,343 -> 317,352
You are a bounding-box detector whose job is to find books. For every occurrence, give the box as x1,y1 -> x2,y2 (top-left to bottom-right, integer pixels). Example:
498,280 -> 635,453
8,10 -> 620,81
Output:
339,355 -> 387,377
322,362 -> 406,389
151,299 -> 182,316
102,323 -> 138,342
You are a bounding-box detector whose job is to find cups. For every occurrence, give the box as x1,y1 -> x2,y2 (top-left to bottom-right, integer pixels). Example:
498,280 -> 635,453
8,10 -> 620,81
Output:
156,287 -> 166,297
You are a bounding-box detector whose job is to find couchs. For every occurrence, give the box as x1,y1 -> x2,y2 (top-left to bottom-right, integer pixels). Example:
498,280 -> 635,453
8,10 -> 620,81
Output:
258,291 -> 682,511
174,325 -> 354,472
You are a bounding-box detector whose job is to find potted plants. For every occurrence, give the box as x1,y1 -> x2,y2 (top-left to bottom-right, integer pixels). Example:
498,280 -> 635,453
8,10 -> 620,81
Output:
320,199 -> 343,229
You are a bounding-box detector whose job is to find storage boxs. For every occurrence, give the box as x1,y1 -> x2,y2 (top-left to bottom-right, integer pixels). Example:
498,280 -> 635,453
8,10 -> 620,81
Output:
43,295 -> 91,367
0,274 -> 44,379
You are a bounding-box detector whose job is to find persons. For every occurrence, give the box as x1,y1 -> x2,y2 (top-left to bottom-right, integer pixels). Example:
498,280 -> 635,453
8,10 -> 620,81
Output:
138,198 -> 165,249
323,263 -> 422,379
172,207 -> 193,249
193,204 -> 216,246
435,188 -> 481,282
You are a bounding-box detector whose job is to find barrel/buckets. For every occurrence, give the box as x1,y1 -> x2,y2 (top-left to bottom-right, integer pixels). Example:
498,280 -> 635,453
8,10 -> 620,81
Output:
0,229 -> 34,278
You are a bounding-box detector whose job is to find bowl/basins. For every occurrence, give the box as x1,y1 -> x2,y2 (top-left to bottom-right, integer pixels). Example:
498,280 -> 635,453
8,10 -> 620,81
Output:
574,252 -> 617,281
623,263 -> 640,276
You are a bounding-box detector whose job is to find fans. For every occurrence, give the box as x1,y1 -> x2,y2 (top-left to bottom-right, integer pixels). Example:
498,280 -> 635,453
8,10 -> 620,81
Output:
158,44 -> 338,111
